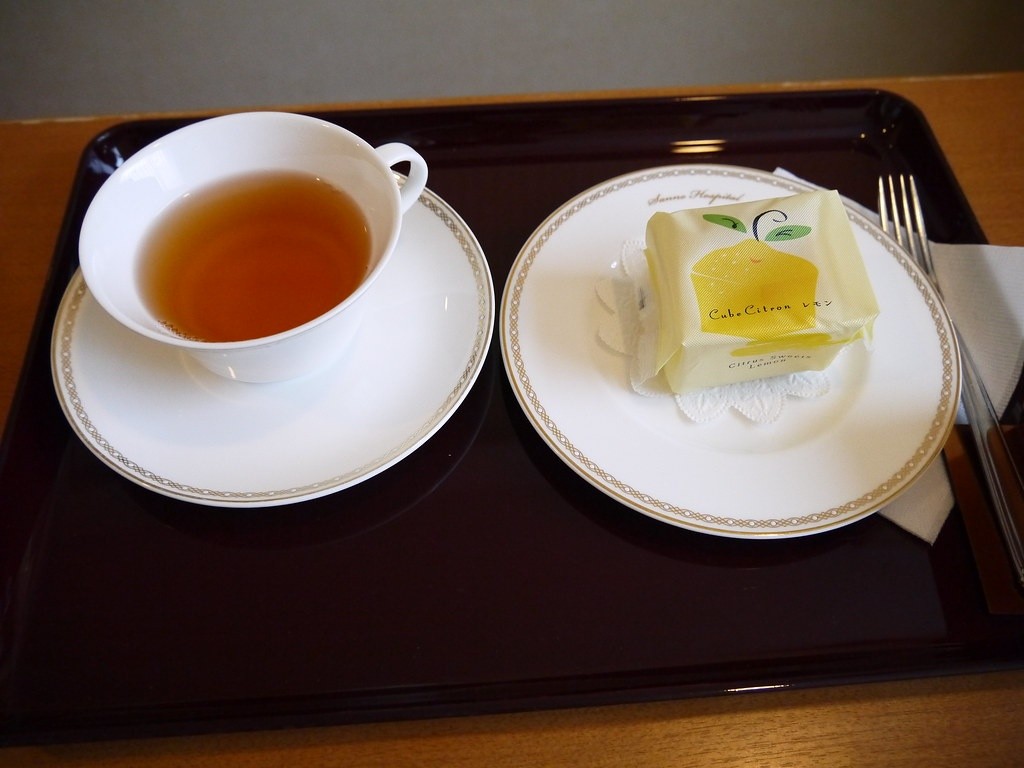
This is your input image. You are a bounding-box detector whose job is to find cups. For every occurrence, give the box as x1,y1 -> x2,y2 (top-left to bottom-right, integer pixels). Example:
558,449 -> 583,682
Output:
79,112 -> 428,386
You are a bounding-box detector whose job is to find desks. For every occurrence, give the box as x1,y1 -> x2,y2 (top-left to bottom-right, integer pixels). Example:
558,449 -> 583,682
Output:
0,69 -> 1024,768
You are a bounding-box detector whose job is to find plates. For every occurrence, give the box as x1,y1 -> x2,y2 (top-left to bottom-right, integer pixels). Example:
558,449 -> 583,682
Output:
50,170 -> 495,508
499,164 -> 962,539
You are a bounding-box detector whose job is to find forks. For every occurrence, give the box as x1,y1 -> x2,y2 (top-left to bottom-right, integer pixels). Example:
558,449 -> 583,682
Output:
877,173 -> 1024,589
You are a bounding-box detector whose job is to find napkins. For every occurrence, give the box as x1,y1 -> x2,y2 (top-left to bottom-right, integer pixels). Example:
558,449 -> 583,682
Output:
770,170 -> 1024,546
